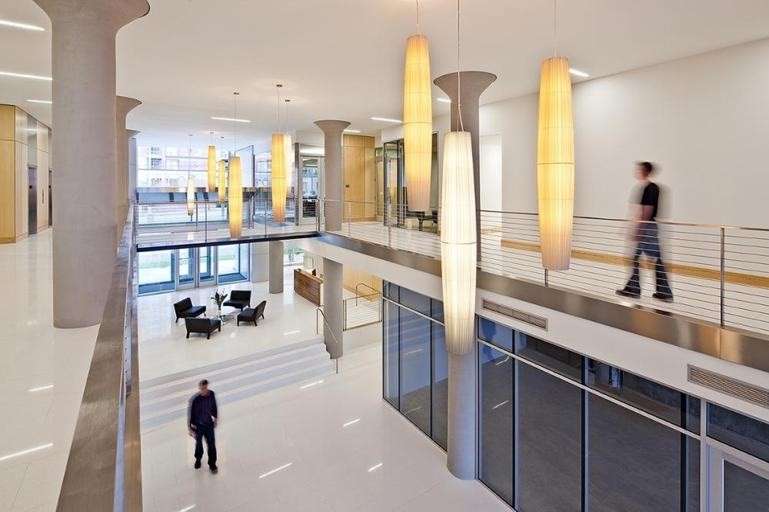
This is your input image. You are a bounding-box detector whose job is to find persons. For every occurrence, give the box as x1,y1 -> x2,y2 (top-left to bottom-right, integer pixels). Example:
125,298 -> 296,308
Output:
187,378 -> 219,475
613,161 -> 674,302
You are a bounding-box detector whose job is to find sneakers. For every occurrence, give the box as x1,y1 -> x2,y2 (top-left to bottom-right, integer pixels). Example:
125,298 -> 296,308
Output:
208,461 -> 217,472
614,288 -> 639,298
194,459 -> 200,469
651,292 -> 673,302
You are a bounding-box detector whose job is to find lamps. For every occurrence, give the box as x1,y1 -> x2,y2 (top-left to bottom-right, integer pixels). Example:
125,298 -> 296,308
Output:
217,135 -> 226,206
271,84 -> 288,224
207,130 -> 216,194
284,97 -> 294,199
532,4 -> 574,275
402,4 -> 436,215
227,91 -> 244,239
187,134 -> 195,216
440,3 -> 477,353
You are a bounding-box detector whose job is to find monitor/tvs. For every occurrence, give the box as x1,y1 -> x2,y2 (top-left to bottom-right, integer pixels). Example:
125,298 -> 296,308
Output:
312,269 -> 316,276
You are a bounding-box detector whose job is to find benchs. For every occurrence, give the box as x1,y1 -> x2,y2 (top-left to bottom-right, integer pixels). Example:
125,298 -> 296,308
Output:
174,298 -> 206,323
